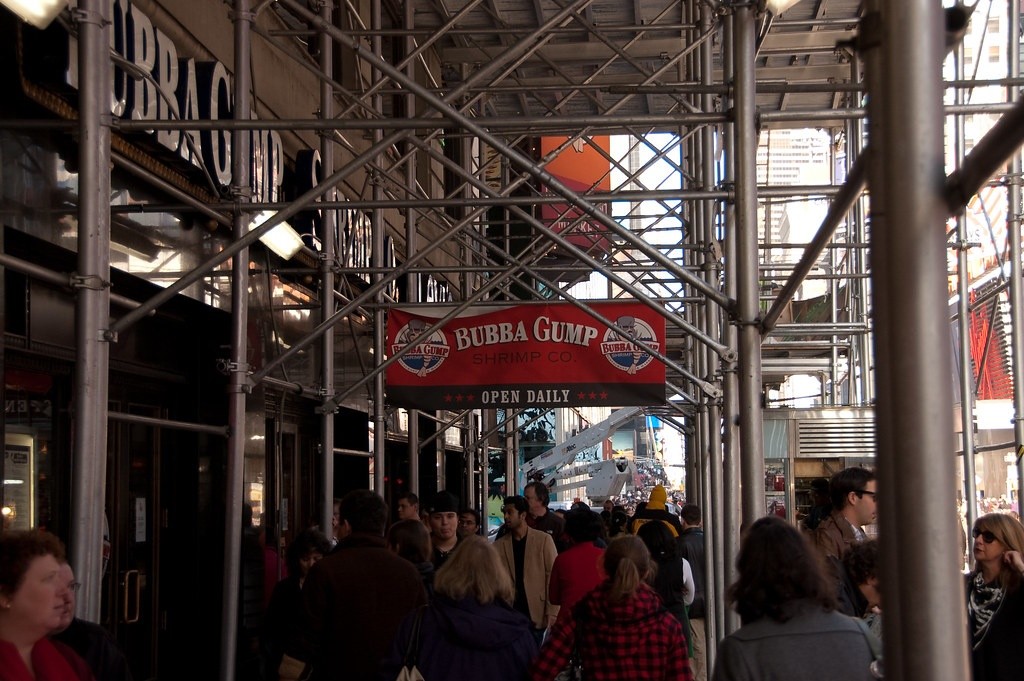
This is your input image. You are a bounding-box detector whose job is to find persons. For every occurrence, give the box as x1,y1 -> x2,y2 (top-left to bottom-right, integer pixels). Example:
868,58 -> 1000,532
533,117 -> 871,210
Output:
382,481 -> 708,681
0,527 -> 134,681
964,514 -> 1024,681
238,489 -> 479,681
957,494 -> 1024,571
798,467 -> 884,681
710,516 -> 877,681
528,535 -> 693,681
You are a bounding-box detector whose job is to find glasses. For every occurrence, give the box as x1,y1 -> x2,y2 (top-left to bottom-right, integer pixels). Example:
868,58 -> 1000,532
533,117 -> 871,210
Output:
973,527 -> 998,544
849,488 -> 877,502
70,582 -> 81,593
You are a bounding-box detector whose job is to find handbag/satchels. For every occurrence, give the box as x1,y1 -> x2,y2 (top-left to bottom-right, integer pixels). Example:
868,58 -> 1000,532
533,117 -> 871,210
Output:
553,599 -> 588,681
396,612 -> 426,681
